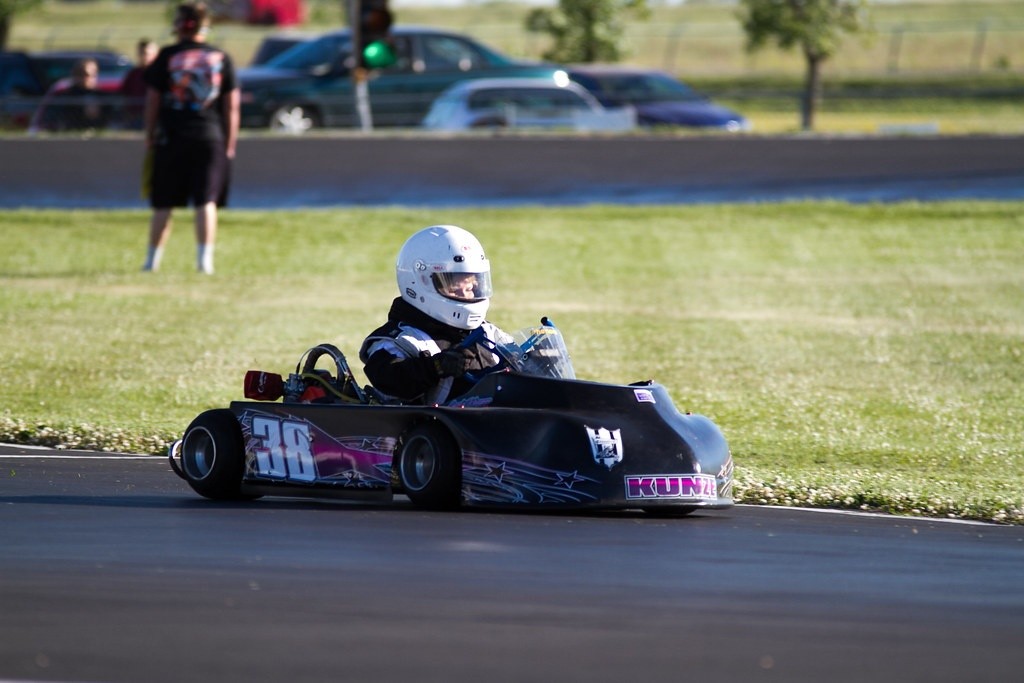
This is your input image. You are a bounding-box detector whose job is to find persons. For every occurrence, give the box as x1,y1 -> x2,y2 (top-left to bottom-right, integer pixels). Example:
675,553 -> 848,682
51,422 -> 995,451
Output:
358,222 -> 521,405
37,35 -> 162,94
138,2 -> 239,276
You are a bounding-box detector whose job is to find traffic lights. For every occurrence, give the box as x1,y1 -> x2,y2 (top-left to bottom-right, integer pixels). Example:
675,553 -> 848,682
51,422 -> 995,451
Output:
354,0 -> 398,72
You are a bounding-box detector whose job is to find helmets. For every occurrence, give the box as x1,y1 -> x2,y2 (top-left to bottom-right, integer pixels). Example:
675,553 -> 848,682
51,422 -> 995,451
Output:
396,225 -> 492,329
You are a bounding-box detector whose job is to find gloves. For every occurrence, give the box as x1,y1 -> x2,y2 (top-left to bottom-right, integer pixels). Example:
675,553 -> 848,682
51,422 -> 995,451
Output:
529,338 -> 558,370
433,344 -> 475,378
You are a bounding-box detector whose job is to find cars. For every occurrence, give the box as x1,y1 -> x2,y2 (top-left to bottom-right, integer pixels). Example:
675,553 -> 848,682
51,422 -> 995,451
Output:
29,71 -> 129,131
419,70 -> 746,132
3,51 -> 149,130
236,28 -> 567,133
